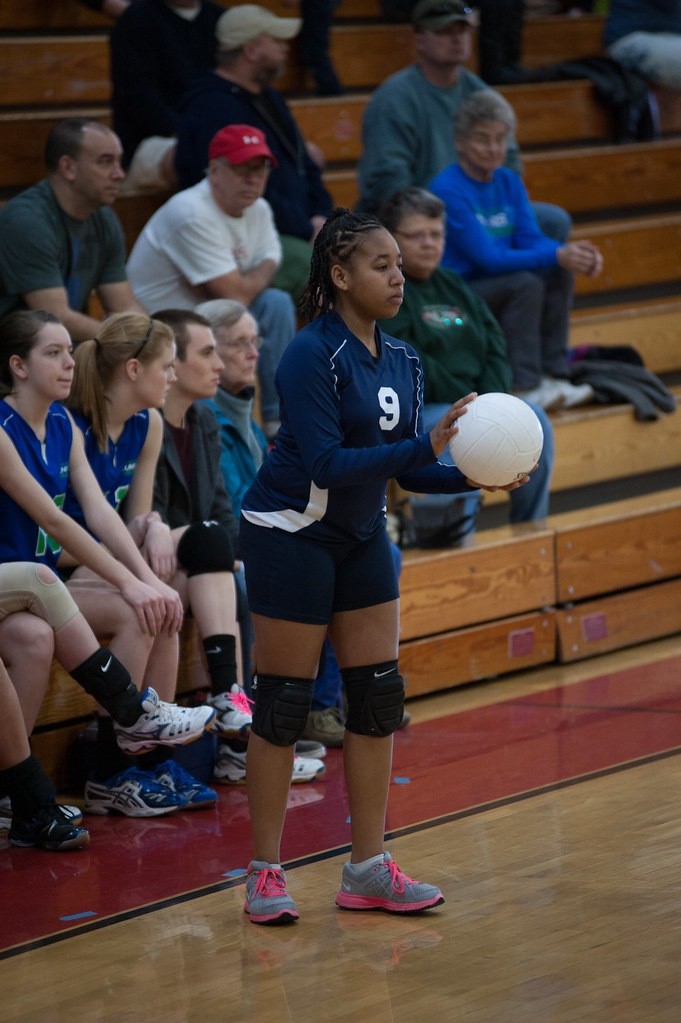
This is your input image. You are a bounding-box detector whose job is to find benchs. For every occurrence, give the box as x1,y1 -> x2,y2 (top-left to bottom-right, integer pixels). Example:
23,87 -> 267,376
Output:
0,0 -> 681,786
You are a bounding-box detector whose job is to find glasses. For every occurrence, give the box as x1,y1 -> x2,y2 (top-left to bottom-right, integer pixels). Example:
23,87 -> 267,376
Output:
219,160 -> 270,178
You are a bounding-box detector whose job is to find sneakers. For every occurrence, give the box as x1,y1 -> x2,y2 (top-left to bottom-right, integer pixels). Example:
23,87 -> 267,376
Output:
161,815 -> 221,854
202,683 -> 255,741
114,687 -> 218,755
153,759 -> 218,808
8,803 -> 90,850
343,693 -> 410,728
84,766 -> 189,817
295,738 -> 327,758
335,907 -> 443,972
0,797 -> 82,826
303,708 -> 346,746
217,785 -> 326,827
511,387 -> 564,412
214,746 -> 325,784
240,920 -> 298,969
334,851 -> 445,912
87,816 -> 177,857
244,860 -> 299,924
540,375 -> 595,407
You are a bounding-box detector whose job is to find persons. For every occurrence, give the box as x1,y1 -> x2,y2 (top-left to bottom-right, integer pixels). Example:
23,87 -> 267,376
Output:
378,184 -> 553,549
361,0 -> 572,242
127,125 -> 295,449
172,4 -> 333,308
427,93 -> 604,412
379,0 -> 557,89
295,0 -> 341,98
0,299 -> 410,820
109,0 -> 226,174
236,206 -> 539,927
606,0 -> 681,95
0,117 -> 142,343
0,561 -> 217,851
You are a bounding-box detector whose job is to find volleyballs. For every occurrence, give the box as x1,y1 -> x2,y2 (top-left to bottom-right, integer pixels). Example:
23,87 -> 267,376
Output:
449,392 -> 544,488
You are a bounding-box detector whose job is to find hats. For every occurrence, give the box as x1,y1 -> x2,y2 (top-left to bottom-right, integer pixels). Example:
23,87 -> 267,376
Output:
217,4 -> 303,51
209,124 -> 277,169
412,0 -> 476,32
